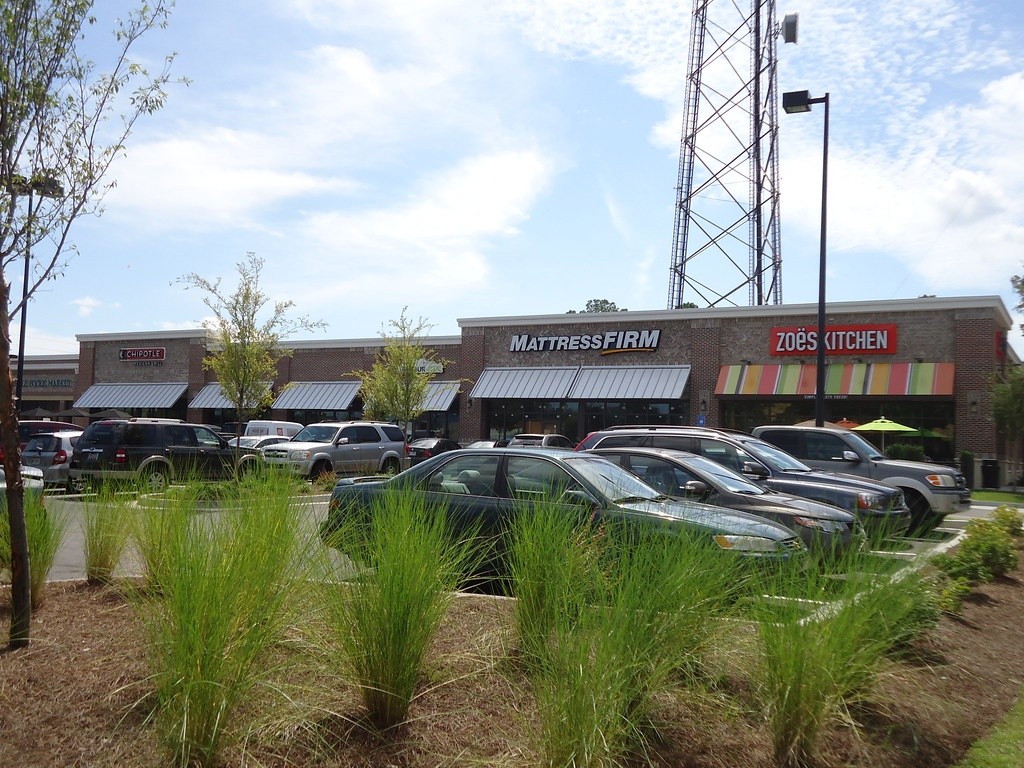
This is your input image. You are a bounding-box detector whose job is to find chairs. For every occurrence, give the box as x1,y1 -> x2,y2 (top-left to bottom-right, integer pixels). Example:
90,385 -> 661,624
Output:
646,462 -> 680,496
430,469 -> 480,497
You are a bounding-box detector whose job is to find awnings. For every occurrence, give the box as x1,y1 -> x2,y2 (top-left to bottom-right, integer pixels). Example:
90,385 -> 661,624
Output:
714,362 -> 954,403
469,364 -> 691,399
270,380 -> 461,411
188,380 -> 274,409
72,381 -> 188,408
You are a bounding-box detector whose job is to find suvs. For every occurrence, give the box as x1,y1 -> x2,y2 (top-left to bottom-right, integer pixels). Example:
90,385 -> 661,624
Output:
21,431 -> 92,493
70,416 -> 265,495
263,419 -> 409,485
574,424 -> 909,545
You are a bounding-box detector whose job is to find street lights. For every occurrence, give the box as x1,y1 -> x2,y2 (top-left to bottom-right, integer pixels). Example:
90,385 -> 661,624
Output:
783,90 -> 829,427
0,172 -> 67,429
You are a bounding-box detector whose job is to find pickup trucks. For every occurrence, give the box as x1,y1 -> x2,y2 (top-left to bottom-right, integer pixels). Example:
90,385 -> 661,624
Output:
705,423 -> 974,537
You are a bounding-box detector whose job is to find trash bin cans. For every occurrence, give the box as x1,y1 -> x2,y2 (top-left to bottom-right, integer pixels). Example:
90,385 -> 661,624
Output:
981,460 -> 1000,488
959,451 -> 974,487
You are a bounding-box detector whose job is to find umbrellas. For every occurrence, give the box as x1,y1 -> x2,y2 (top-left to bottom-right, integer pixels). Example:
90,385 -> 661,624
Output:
794,416 -> 918,454
20,406 -> 132,424
898,426 -> 949,437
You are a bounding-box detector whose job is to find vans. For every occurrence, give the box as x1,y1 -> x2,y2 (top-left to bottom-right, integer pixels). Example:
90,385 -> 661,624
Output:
508,434 -> 573,449
219,423 -> 249,449
245,419 -> 303,439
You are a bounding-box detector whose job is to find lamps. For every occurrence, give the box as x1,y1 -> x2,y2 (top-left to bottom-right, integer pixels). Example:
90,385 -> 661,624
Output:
970,402 -> 977,413
852,358 -> 862,363
915,357 -> 923,363
795,359 -> 805,365
740,357 -> 751,365
700,400 -> 706,411
467,400 -> 472,409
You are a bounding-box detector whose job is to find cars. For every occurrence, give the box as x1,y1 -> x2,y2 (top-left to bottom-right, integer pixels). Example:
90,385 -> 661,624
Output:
228,434 -> 291,450
465,440 -> 509,449
510,444 -> 868,571
408,438 -> 462,466
0,461 -> 48,527
320,447 -> 811,612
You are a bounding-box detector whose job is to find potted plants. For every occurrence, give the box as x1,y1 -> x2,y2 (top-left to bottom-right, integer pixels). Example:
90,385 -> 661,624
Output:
959,447 -> 976,488
